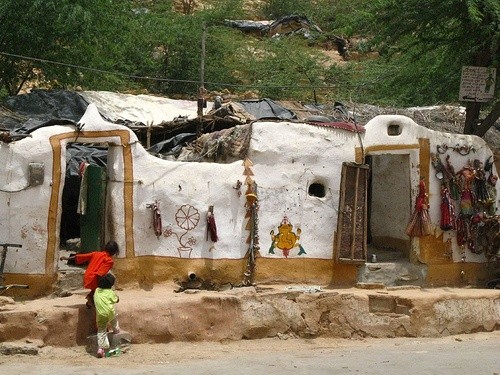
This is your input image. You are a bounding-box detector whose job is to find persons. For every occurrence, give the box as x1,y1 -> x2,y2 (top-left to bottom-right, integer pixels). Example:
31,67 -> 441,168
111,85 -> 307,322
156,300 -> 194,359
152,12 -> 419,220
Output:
93,272 -> 121,358
59,241 -> 120,310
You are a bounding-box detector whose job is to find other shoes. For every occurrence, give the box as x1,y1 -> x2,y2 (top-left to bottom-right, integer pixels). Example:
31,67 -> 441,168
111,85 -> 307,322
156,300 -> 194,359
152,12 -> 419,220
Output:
86,294 -> 93,307
112,328 -> 120,333
96,348 -> 105,358
